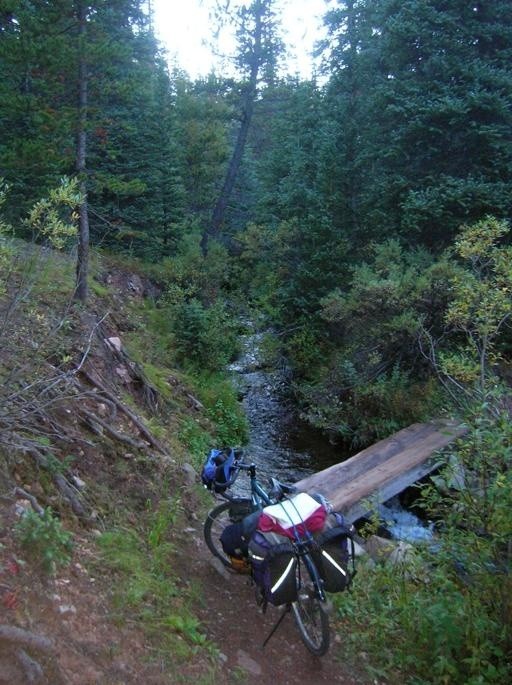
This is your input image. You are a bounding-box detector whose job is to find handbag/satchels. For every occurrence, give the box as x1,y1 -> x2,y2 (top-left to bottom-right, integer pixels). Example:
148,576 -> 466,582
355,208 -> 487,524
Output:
310,494 -> 362,593
219,525 -> 249,559
247,529 -> 299,613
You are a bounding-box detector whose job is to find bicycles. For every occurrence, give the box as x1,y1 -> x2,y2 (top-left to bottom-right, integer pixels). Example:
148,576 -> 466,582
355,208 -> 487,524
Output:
201,445 -> 334,657
